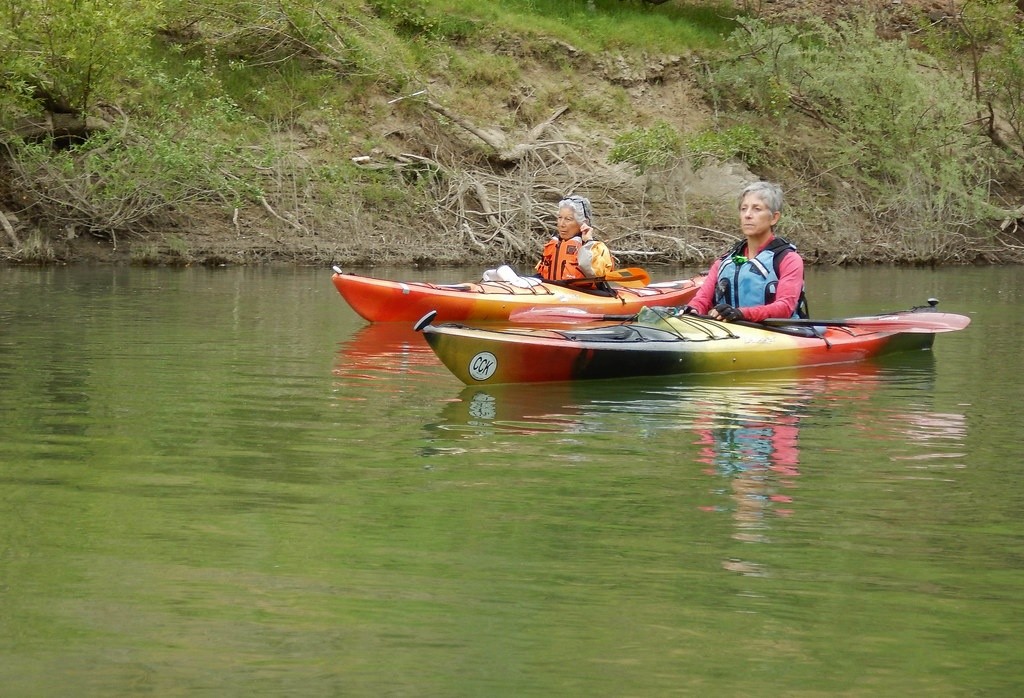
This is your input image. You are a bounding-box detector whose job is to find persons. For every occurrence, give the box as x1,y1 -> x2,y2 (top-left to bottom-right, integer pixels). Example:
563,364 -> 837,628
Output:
684,181 -> 803,322
535,195 -> 612,279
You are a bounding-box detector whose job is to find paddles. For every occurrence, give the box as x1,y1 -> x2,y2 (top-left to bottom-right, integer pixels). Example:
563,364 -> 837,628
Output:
549,268 -> 652,292
510,305 -> 972,334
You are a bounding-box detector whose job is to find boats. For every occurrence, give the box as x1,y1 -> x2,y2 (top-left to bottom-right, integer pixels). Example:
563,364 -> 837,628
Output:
413,295 -> 973,383
329,263 -> 716,323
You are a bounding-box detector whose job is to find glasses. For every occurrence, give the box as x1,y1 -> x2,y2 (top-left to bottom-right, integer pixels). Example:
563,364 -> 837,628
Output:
564,197 -> 587,218
